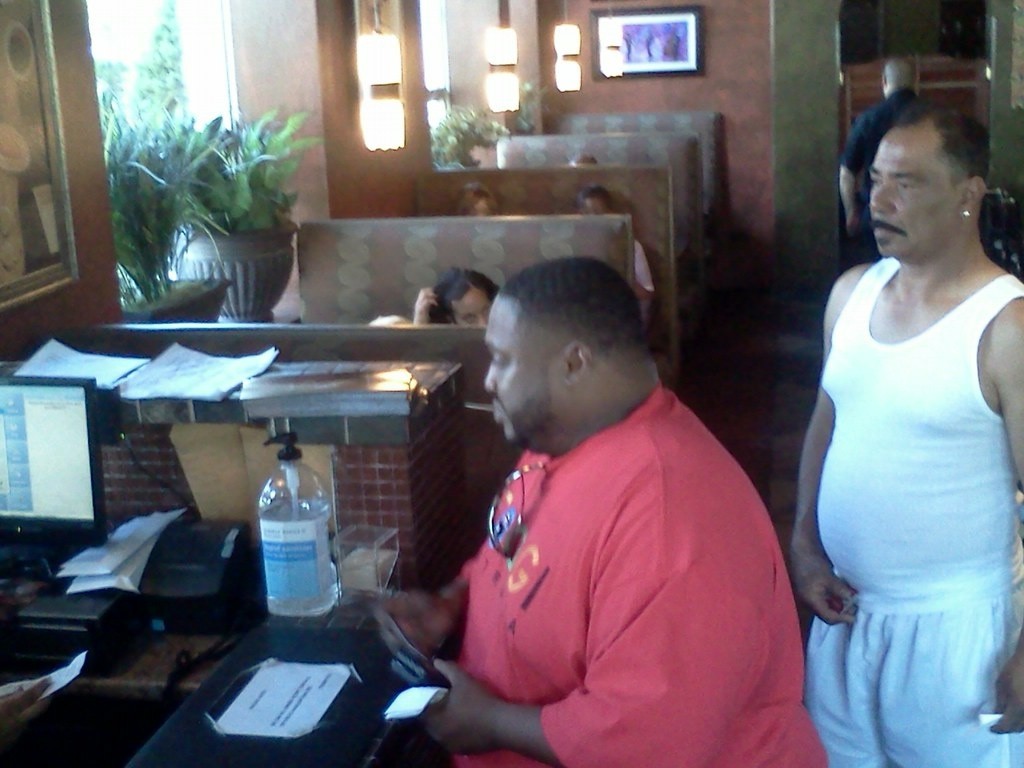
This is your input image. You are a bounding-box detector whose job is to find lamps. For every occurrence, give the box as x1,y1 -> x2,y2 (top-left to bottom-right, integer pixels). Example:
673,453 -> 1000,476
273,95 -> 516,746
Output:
553,24 -> 582,91
355,33 -> 405,150
486,27 -> 519,112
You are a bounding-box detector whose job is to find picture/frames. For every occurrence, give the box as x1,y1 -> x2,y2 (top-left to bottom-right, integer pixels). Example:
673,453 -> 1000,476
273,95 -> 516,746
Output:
1,1 -> 81,313
588,5 -> 701,82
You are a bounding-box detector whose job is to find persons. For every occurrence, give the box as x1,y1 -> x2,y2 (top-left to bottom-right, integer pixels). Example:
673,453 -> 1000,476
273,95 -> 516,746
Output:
408,264 -> 500,329
563,154 -> 600,167
450,180 -> 501,221
369,255 -> 830,768
837,53 -> 925,265
783,100 -> 1022,768
570,181 -> 655,332
0,674 -> 54,757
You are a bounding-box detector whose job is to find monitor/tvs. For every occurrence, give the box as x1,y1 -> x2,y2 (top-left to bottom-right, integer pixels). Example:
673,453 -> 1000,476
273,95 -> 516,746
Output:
1,376 -> 107,545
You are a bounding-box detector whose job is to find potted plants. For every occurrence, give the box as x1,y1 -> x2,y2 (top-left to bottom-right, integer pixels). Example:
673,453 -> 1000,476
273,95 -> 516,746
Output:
99,92 -> 230,324
176,112 -> 324,323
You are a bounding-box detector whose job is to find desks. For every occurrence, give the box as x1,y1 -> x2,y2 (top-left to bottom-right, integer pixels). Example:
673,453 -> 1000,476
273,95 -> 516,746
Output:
0,589 -> 439,768
0,361 -> 465,588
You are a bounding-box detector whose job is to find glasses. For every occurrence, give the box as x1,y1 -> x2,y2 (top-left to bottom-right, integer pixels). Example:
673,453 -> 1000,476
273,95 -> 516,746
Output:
488,462 -> 543,557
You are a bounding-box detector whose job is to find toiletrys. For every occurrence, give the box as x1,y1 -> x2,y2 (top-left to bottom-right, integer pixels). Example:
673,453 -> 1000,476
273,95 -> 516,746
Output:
255,429 -> 343,619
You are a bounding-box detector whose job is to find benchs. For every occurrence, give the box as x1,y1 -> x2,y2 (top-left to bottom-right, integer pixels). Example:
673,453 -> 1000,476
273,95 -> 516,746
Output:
497,132 -> 707,333
555,110 -> 725,282
297,214 -> 634,325
416,165 -> 679,379
32,326 -> 524,544
